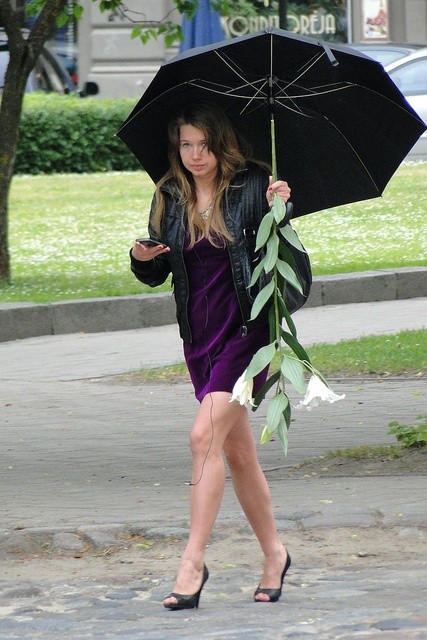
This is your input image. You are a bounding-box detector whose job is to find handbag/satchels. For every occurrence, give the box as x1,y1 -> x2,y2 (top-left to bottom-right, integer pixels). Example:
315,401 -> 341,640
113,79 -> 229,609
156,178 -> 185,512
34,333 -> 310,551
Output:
262,220 -> 314,315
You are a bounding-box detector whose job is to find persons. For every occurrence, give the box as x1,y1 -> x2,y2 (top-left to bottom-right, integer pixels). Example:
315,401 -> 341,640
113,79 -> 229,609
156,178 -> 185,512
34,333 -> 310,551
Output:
125,109 -> 311,610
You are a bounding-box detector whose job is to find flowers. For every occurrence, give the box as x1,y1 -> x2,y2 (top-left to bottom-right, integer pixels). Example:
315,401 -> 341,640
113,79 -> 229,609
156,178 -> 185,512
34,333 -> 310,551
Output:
230,118 -> 345,455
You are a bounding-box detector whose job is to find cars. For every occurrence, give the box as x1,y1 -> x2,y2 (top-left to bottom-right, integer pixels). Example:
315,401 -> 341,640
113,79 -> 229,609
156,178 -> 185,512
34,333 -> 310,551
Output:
0,38 -> 98,98
341,43 -> 427,69
382,47 -> 426,161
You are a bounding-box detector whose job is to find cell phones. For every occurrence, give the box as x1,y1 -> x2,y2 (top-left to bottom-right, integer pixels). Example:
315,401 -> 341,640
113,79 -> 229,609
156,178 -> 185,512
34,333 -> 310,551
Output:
135,238 -> 168,252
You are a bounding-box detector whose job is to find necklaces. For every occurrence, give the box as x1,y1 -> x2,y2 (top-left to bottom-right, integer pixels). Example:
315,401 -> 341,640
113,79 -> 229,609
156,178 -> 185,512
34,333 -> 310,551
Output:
198,201 -> 213,221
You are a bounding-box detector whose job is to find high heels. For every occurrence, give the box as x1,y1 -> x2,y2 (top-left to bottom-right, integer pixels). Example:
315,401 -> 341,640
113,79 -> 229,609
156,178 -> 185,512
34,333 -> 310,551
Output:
253,549 -> 291,603
162,560 -> 209,610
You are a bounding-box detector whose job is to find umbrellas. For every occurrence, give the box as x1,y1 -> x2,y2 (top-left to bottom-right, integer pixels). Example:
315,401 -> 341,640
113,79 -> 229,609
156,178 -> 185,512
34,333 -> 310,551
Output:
115,25 -> 426,230
